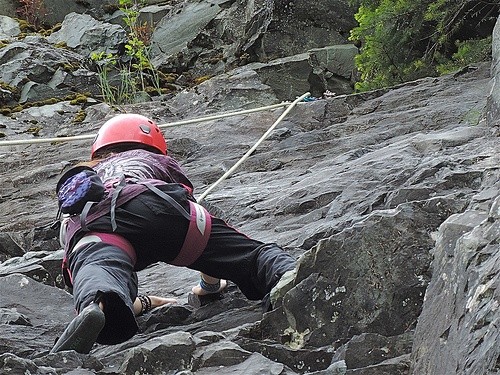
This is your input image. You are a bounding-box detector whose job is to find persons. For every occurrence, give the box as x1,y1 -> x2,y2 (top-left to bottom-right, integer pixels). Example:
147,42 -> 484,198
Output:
52,114 -> 306,354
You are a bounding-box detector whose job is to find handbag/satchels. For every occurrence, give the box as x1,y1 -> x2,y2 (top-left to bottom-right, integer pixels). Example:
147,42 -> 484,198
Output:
55,165 -> 103,215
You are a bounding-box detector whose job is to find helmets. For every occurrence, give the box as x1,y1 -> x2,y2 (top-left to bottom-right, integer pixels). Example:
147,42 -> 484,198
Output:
92,112 -> 167,158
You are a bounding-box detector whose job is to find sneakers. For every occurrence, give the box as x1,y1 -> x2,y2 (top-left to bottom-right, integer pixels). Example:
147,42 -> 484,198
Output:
49,305 -> 104,356
